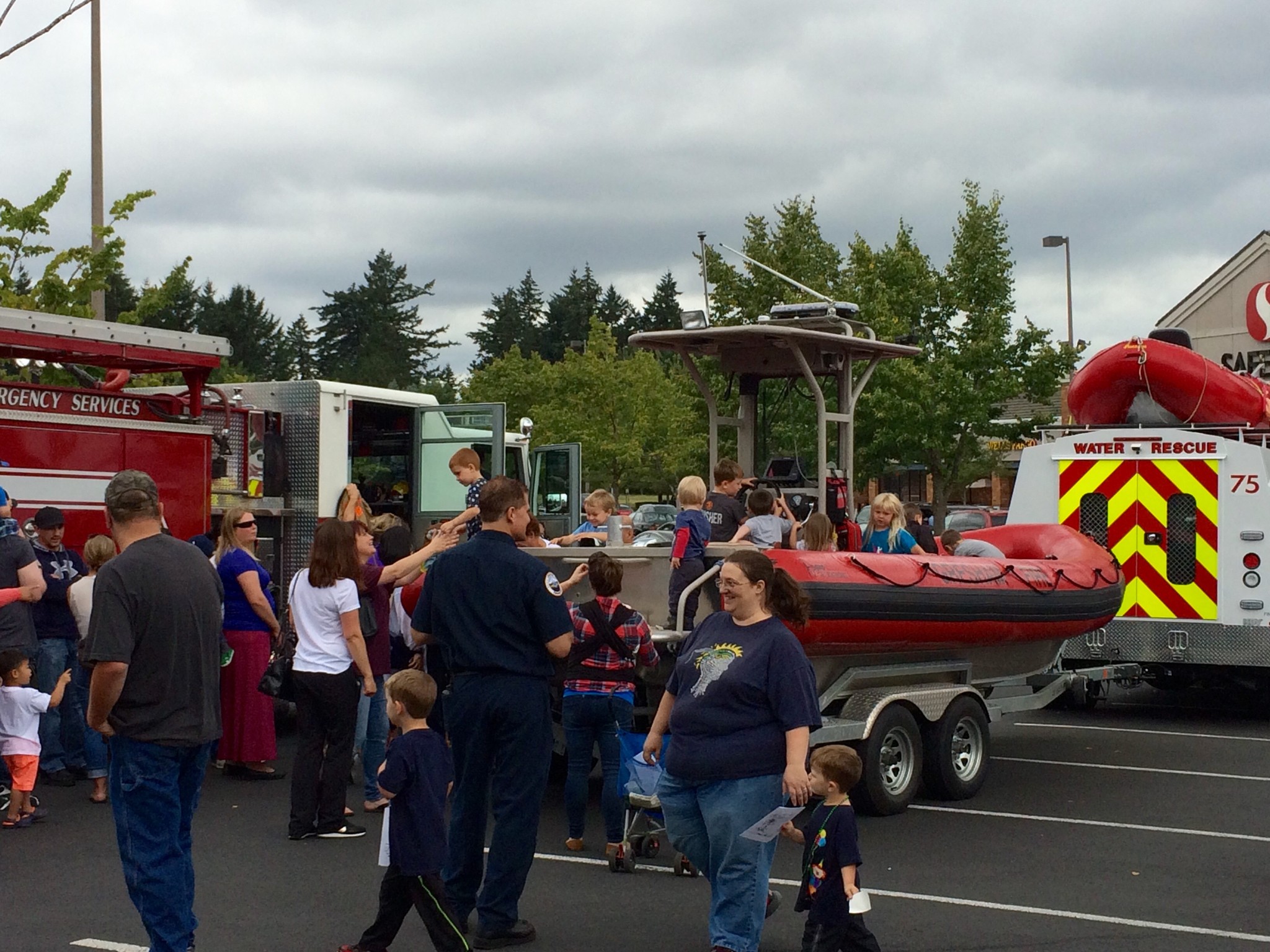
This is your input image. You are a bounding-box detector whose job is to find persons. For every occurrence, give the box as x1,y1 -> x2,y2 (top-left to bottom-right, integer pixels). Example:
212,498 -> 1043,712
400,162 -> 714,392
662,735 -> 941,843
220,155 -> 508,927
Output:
214,506 -> 286,781
902,503 -> 939,555
515,488 -> 635,547
940,530 -> 1006,559
788,512 -> 836,551
440,448 -> 488,542
862,493 -> 931,555
288,518 -> 377,840
727,489 -> 796,548
343,519 -> 459,815
700,457 -> 758,542
780,744 -> 881,952
557,551 -> 660,854
82,468 -> 223,952
337,669 -> 474,952
0,488 -> 217,826
410,475 -> 575,950
643,551 -> 822,952
655,476 -> 712,630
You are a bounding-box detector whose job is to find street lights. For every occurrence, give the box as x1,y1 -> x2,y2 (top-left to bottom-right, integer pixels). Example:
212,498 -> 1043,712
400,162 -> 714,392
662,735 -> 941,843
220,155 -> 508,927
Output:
1042,237 -> 1073,384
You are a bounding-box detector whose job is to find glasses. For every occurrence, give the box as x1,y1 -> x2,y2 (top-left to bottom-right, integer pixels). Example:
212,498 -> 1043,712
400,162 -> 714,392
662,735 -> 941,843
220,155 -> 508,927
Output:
235,519 -> 257,528
715,578 -> 752,587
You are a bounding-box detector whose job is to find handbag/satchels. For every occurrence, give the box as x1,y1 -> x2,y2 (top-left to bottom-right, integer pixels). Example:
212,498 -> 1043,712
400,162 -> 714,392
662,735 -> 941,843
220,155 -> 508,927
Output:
794,871 -> 813,912
271,605 -> 296,654
257,652 -> 301,702
357,594 -> 378,637
377,805 -> 392,867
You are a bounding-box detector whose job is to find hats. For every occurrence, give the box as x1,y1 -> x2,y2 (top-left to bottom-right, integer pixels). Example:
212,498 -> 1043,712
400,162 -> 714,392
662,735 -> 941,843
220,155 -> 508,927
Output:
187,536 -> 215,556
104,470 -> 158,507
35,506 -> 63,528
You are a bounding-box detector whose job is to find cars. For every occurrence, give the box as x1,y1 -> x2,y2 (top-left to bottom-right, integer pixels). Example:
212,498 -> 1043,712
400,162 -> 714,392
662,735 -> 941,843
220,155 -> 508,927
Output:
582,493 -> 677,547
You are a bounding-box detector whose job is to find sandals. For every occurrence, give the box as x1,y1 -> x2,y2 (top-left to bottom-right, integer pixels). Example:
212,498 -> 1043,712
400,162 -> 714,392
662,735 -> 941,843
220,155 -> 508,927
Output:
2,814 -> 30,828
19,808 -> 48,821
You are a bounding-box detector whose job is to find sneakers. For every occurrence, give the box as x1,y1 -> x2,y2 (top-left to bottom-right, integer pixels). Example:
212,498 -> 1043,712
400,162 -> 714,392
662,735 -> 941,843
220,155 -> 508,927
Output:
288,817 -> 366,839
220,648 -> 234,667
336,943 -> 366,952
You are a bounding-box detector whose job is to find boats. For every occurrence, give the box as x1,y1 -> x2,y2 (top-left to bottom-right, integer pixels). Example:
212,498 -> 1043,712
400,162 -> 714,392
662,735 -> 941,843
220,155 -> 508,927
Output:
404,522 -> 1127,669
1066,337 -> 1269,429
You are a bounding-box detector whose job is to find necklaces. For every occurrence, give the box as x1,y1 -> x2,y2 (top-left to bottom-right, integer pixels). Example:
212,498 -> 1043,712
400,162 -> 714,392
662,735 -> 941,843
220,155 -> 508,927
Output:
801,795 -> 850,879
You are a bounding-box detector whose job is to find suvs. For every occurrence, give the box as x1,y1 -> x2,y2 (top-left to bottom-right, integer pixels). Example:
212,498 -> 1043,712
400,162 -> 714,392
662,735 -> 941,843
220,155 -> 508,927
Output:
856,501 -> 1009,534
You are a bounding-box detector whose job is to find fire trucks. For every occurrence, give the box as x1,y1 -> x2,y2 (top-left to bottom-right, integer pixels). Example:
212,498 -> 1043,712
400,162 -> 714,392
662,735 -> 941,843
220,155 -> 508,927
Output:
0,307 -> 583,729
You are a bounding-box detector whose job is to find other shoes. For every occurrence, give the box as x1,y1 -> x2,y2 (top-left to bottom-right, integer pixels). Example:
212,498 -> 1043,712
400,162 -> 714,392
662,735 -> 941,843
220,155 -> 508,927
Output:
565,837 -> 584,851
0,784 -> 11,810
213,759 -> 286,780
349,752 -> 359,783
472,920 -> 536,949
28,767 -> 107,806
764,888 -> 782,918
344,803 -> 389,816
655,617 -> 693,633
606,843 -> 620,854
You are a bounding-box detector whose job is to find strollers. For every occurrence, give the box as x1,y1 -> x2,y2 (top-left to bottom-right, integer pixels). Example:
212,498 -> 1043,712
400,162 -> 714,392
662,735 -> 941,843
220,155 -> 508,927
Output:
605,685 -> 700,878
